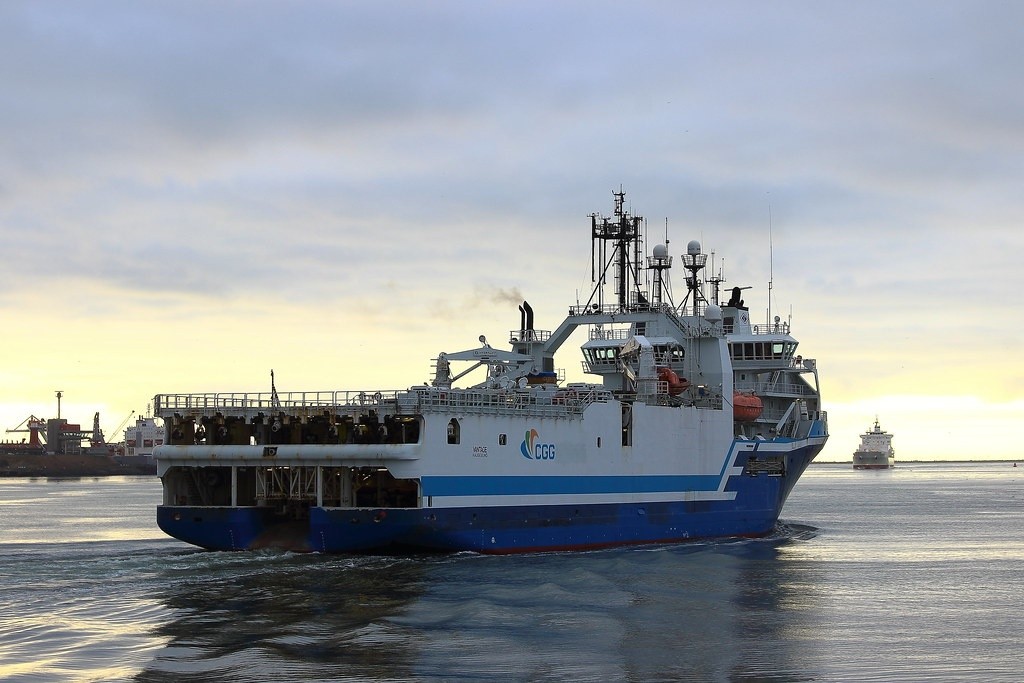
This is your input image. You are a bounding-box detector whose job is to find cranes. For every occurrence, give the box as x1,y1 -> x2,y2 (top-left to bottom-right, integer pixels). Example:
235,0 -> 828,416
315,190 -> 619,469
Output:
9,410 -> 136,444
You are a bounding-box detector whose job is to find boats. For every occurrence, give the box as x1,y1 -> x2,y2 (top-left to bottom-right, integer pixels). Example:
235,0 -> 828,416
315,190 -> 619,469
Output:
112,453 -> 156,476
853,415 -> 896,470
657,368 -> 689,395
152,180 -> 829,554
733,390 -> 764,422
